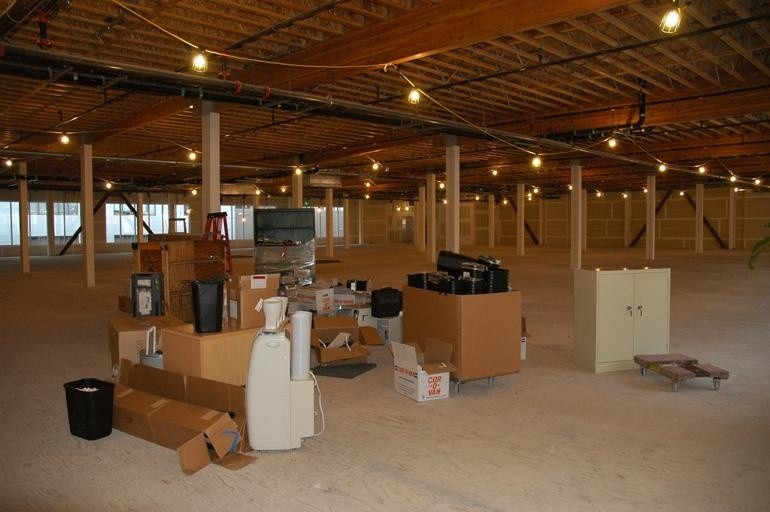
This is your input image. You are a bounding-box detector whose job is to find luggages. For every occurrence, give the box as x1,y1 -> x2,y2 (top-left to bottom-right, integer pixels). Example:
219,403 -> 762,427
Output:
140,326 -> 163,370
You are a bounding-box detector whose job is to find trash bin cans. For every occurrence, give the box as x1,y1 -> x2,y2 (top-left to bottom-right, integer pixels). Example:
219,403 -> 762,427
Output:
63,378 -> 115,440
139,348 -> 164,368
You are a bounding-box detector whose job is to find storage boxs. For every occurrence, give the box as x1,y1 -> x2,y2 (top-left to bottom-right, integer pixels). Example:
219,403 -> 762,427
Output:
285,255 -> 520,402
111,273 -> 290,476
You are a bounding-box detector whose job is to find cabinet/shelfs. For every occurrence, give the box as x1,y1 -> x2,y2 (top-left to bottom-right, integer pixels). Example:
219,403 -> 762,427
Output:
572,266 -> 670,373
131,233 -> 226,324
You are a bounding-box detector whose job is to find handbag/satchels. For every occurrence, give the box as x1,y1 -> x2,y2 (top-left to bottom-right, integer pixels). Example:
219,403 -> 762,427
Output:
371,287 -> 401,318
